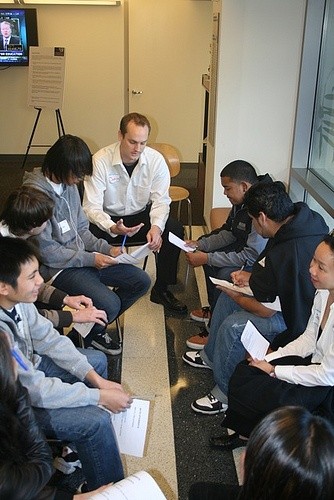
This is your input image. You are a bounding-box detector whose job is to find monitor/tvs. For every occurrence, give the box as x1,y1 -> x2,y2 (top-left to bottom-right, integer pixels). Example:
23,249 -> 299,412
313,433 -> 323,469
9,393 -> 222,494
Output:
0,8 -> 38,67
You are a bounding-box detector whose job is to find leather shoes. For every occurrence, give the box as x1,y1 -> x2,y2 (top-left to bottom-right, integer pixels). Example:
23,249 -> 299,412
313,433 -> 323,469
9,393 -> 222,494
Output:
150,286 -> 188,318
209,432 -> 248,450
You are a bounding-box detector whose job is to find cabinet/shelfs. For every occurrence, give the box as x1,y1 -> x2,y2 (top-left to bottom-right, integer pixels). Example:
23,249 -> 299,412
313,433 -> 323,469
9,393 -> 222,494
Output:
196,74 -> 210,210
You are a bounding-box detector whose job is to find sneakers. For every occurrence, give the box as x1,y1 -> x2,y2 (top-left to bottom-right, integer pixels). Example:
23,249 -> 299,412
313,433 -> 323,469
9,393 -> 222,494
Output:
190,307 -> 212,322
191,393 -> 228,414
76,482 -> 89,494
186,329 -> 209,350
90,331 -> 121,355
53,447 -> 82,474
182,350 -> 212,369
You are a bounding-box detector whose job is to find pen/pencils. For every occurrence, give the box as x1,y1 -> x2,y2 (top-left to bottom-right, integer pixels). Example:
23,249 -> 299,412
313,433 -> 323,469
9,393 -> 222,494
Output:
121,235 -> 126,253
10,350 -> 28,371
192,250 -> 196,253
233,259 -> 248,285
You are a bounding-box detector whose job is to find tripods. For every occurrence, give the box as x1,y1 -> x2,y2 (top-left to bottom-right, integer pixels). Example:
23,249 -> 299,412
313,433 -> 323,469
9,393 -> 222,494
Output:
21,107 -> 66,168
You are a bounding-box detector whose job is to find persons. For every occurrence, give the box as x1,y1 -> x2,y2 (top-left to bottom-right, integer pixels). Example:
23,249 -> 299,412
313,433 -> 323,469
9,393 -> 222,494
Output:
82,112 -> 187,313
188,407 -> 334,500
22,133 -> 151,355
180,179 -> 331,414
0,21 -> 20,50
0,185 -> 109,333
0,332 -> 113,500
0,236 -> 132,493
186,160 -> 274,350
208,236 -> 334,451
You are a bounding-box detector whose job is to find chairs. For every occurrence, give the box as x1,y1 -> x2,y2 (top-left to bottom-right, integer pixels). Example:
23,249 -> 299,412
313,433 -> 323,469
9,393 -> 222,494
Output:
146,143 -> 192,226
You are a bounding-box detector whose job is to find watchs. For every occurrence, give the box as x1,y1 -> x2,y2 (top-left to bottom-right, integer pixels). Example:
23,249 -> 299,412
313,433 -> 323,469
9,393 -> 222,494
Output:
269,366 -> 276,378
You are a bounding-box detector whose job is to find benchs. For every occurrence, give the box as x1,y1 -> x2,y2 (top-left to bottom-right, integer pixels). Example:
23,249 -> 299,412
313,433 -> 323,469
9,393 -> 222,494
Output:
210,207 -> 232,230
